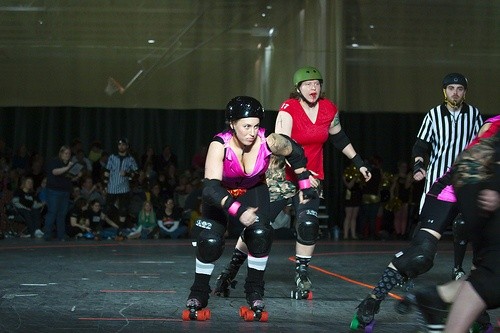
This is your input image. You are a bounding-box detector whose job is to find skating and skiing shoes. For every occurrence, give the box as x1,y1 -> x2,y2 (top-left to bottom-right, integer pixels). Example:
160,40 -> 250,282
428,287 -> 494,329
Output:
396,285 -> 449,333
181,274 -> 211,321
240,268 -> 268,322
214,273 -> 238,298
350,295 -> 383,333
291,273 -> 315,300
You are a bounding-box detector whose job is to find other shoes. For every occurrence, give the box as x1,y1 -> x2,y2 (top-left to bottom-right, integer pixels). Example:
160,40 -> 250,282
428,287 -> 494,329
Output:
352,237 -> 361,241
20,233 -> 31,238
344,237 -> 350,241
451,265 -> 466,281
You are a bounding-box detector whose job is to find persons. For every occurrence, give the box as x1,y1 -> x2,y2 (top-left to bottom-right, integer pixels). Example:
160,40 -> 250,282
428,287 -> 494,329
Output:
343,161 -> 413,241
0,139 -> 208,241
182,96 -> 304,321
444,131 -> 500,333
388,73 -> 483,299
350,115 -> 500,333
213,66 -> 371,299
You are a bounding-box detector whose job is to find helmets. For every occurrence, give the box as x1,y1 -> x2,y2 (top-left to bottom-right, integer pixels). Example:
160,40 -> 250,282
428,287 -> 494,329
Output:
226,97 -> 266,119
115,139 -> 126,145
441,72 -> 469,88
293,66 -> 323,86
